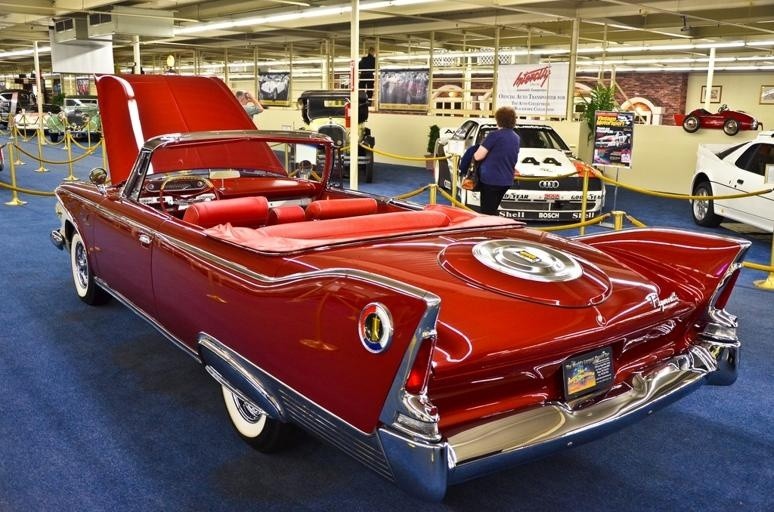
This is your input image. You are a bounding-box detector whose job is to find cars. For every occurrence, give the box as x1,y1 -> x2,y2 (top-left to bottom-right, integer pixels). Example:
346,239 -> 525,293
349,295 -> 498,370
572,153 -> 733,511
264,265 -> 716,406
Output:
260,79 -> 286,99
0,87 -> 101,144
384,80 -> 428,106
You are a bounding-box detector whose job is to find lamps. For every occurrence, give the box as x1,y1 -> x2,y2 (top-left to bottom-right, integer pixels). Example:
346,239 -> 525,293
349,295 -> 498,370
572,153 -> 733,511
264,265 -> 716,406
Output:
163,54 -> 176,76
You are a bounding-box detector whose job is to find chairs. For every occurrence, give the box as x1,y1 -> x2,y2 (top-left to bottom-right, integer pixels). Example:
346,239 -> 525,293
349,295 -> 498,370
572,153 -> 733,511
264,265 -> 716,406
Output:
180,195 -> 451,243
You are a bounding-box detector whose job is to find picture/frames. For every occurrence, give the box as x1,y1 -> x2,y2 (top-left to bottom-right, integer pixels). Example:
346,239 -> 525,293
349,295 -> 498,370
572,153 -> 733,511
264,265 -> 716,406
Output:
758,85 -> 774,105
700,85 -> 722,104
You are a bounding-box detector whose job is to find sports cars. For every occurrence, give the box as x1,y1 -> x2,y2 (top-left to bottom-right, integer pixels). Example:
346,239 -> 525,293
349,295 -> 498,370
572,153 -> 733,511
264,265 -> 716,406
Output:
430,113 -> 608,225
686,130 -> 774,235
672,105 -> 758,136
47,73 -> 757,505
596,131 -> 631,147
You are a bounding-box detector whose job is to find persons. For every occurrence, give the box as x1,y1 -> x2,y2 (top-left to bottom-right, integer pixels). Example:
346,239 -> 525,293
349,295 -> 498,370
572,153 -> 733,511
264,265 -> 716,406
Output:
358,46 -> 380,105
233,90 -> 263,173
473,107 -> 520,219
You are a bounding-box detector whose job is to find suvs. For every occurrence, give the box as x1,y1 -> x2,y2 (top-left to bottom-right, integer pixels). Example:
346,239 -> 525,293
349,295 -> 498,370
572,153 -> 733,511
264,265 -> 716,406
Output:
282,86 -> 376,185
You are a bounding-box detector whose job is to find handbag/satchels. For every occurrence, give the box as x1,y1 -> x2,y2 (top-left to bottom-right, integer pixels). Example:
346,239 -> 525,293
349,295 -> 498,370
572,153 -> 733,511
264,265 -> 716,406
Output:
461,158 -> 479,192
